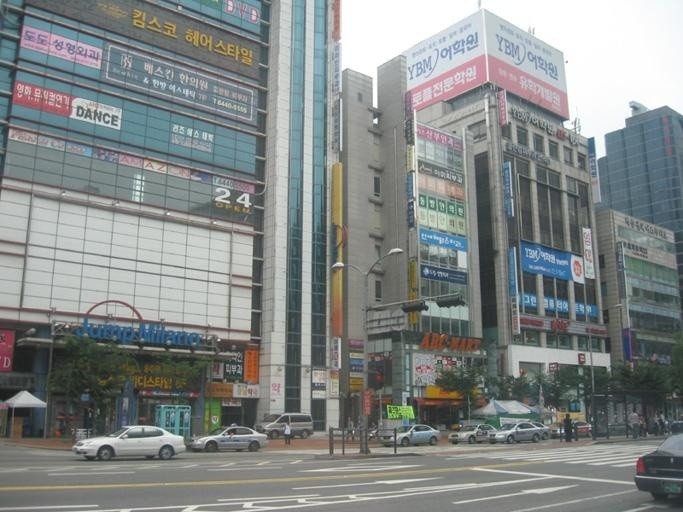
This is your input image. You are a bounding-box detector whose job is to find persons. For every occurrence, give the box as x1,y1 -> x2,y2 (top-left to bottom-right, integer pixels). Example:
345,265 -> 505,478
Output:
638,414 -> 645,438
368,421 -> 377,439
228,429 -> 234,439
282,421 -> 290,446
346,416 -> 354,440
655,410 -> 671,435
629,408 -> 639,440
563,412 -> 573,442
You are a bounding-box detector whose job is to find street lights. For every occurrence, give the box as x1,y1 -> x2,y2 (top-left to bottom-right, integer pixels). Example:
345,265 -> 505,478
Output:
584,304 -> 623,392
331,248 -> 404,453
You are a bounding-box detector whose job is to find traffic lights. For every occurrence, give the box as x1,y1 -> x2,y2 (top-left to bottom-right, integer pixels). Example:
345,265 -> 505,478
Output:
436,296 -> 465,307
402,300 -> 428,312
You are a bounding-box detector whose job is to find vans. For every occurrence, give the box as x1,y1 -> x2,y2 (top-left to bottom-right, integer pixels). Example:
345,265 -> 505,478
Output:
255,412 -> 314,439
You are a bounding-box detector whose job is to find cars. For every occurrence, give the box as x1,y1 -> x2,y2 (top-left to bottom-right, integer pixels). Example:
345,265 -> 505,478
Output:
552,420 -> 592,439
381,424 -> 440,447
190,422 -> 268,452
634,433 -> 683,500
72,425 -> 186,461
448,421 -> 550,444
